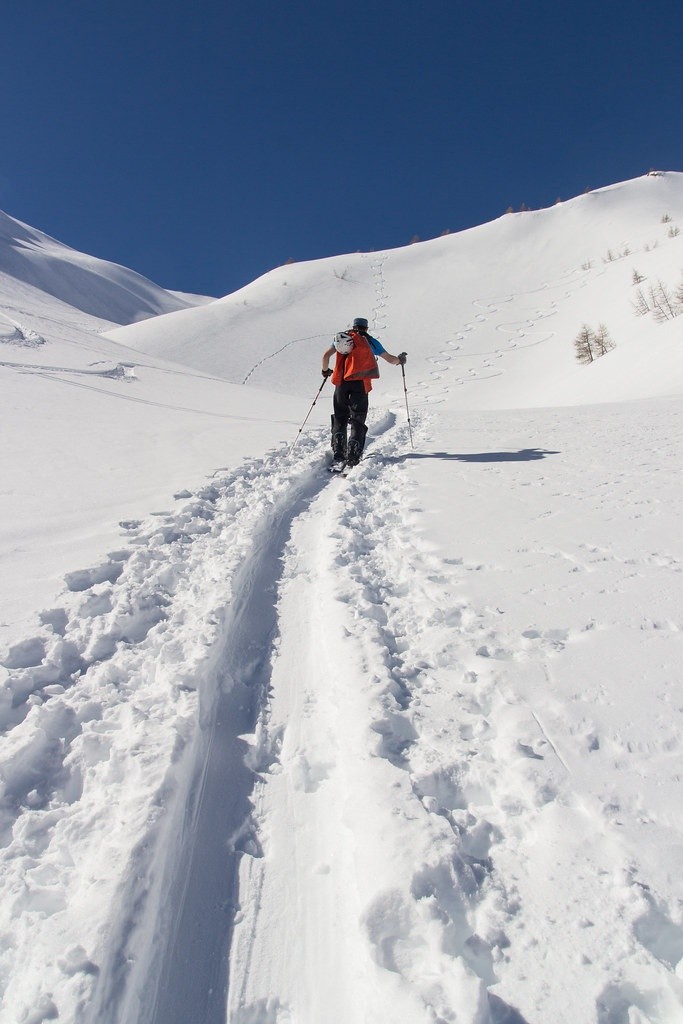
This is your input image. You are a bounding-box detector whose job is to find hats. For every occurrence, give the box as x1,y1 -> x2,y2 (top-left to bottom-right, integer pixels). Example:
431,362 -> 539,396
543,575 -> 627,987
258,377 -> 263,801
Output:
353,318 -> 369,329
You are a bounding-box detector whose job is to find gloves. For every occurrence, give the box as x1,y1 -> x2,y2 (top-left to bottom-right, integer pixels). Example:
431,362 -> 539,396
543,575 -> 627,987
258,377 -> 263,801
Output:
321,368 -> 334,379
398,352 -> 408,366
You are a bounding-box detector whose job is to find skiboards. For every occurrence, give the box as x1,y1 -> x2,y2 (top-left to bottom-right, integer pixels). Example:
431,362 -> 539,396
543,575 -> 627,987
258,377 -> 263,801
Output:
329,459 -> 353,477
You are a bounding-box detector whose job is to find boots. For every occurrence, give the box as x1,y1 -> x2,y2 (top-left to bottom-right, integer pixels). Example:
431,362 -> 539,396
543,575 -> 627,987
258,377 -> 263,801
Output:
327,414 -> 347,474
347,415 -> 368,466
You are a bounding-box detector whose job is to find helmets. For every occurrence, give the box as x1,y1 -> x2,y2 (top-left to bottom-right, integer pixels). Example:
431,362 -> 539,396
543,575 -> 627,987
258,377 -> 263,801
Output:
332,331 -> 355,355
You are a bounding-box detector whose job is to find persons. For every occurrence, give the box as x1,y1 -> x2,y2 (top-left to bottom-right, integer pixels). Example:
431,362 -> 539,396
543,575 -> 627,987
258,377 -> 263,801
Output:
322,318 -> 407,477
